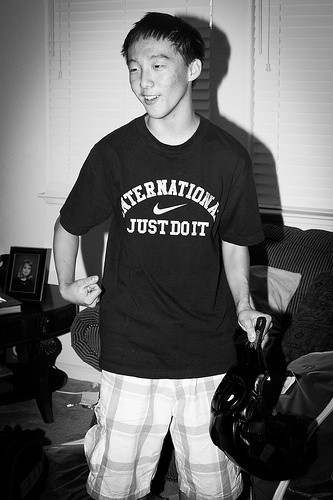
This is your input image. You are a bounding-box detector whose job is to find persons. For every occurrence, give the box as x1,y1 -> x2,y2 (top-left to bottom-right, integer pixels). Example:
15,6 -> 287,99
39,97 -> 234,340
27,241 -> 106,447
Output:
10,259 -> 35,291
51,11 -> 273,500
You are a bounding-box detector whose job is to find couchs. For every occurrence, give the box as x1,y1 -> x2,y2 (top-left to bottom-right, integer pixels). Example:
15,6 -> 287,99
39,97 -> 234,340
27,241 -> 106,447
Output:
71,222 -> 333,500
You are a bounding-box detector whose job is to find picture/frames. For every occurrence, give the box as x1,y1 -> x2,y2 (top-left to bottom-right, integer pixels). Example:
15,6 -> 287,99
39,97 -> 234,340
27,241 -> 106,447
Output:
5,246 -> 51,302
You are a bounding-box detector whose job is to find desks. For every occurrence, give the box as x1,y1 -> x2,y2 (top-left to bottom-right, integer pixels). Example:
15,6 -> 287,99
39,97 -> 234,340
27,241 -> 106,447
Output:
0,284 -> 78,424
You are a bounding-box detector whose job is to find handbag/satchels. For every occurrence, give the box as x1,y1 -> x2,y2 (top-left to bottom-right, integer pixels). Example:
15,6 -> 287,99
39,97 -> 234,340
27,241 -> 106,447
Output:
208,317 -> 309,481
